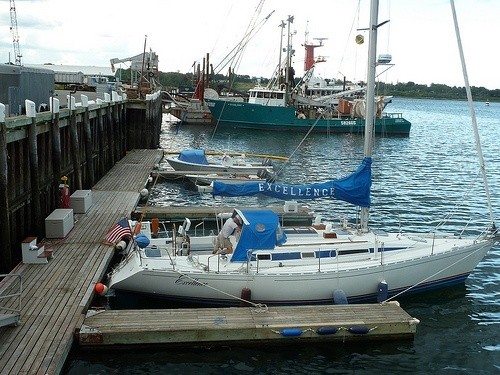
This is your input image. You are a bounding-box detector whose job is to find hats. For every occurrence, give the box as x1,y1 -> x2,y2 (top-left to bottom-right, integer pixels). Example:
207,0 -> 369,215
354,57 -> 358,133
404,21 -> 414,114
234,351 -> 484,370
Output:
235,214 -> 243,224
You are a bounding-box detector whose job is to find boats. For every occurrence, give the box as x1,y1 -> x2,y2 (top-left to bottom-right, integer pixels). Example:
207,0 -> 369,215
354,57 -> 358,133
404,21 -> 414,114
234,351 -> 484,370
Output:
110,0 -> 497,305
154,148 -> 287,188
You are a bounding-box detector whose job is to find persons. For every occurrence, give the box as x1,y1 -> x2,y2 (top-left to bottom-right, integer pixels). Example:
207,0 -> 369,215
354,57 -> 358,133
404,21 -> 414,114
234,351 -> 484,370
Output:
212,214 -> 242,254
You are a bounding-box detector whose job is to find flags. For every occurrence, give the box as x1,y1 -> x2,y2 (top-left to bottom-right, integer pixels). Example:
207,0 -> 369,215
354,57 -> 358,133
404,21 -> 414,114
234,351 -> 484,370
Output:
103,216 -> 132,244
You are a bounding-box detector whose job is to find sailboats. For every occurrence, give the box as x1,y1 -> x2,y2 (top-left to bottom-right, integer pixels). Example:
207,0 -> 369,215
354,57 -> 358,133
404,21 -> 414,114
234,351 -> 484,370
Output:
206,16 -> 418,139
170,50 -> 219,129
